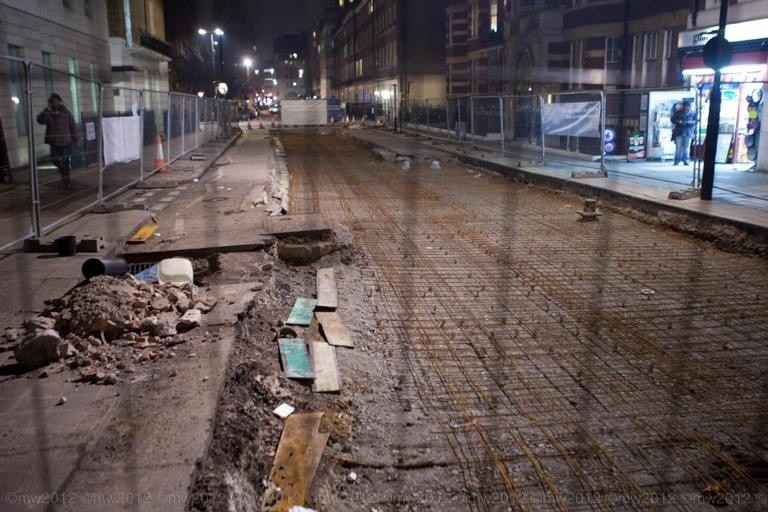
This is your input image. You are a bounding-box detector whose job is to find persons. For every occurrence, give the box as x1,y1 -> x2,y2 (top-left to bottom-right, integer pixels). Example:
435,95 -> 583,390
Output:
38,94 -> 81,190
671,101 -> 697,165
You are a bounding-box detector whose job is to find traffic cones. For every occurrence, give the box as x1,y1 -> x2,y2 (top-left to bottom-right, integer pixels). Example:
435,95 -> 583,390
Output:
151,133 -> 171,174
246,117 -> 275,129
329,114 -> 366,127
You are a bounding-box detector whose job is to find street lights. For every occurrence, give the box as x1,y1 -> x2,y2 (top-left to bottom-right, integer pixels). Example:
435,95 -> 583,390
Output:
218,30 -> 223,97
244,58 -> 251,77
198,26 -> 223,98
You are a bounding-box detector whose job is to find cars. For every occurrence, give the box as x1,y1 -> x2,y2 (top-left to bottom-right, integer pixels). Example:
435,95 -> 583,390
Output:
269,104 -> 279,112
